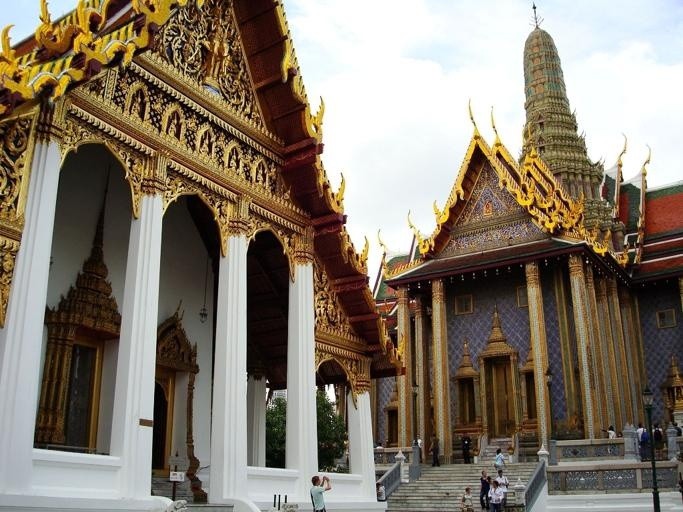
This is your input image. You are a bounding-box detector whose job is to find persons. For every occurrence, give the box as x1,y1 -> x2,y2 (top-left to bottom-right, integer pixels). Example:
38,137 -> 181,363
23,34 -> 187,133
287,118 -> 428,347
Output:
429,432 -> 440,467
461,448 -> 509,512
461,432 -> 472,464
623,419 -> 682,462
376,443 -> 383,448
607,424 -> 617,454
310,476 -> 331,512
414,434 -> 423,463
118,18 -> 291,202
377,482 -> 386,501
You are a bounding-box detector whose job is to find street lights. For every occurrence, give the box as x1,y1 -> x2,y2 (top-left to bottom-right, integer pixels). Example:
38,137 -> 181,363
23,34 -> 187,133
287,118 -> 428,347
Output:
642,382 -> 661,512
412,379 -> 420,446
543,367 -> 556,441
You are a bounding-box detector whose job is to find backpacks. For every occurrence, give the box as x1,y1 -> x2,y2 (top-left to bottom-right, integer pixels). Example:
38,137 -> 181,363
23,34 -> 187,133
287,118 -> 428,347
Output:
654,428 -> 661,439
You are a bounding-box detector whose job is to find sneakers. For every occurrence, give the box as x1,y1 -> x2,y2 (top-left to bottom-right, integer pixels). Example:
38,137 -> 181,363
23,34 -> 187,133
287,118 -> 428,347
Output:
641,456 -> 679,462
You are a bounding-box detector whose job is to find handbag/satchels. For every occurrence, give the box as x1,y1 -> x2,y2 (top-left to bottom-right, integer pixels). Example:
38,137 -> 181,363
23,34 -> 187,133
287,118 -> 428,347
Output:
493,461 -> 504,467
641,429 -> 649,441
